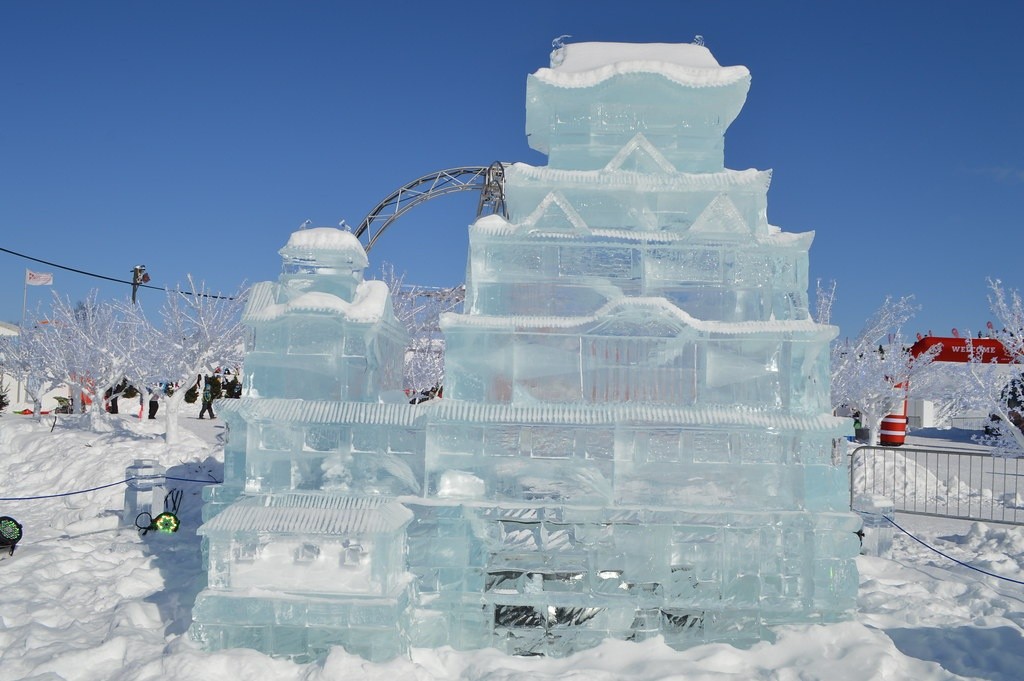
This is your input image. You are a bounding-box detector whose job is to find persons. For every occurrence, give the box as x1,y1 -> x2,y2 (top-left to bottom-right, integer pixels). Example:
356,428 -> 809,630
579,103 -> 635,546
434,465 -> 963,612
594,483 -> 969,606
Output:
109,366 -> 230,419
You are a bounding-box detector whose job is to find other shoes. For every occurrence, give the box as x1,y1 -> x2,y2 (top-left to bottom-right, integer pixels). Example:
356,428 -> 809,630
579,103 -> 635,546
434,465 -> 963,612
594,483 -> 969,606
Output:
199,416 -> 206,419
210,416 -> 217,419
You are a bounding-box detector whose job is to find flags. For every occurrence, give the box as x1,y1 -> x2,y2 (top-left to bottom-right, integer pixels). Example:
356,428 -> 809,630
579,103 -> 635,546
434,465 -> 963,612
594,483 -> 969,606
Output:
26,269 -> 53,286
